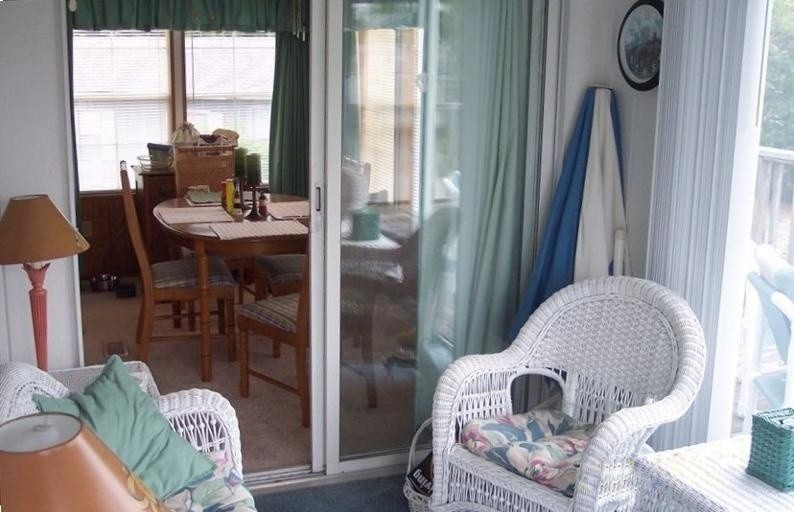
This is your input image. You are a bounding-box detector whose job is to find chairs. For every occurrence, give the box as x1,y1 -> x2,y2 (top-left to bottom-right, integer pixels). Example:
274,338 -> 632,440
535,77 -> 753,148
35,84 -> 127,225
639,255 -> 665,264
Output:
239,237 -> 385,428
1,352 -> 257,512
119,160 -> 239,365
427,271 -> 714,512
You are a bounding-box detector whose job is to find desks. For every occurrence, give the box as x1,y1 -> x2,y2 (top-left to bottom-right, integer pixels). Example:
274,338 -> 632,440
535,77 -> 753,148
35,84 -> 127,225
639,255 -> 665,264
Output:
629,426 -> 794,512
130,162 -> 176,301
153,189 -> 364,383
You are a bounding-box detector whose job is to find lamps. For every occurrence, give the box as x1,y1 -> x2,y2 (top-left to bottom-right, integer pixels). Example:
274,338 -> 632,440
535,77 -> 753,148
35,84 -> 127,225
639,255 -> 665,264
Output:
0,191 -> 91,372
1,411 -> 177,512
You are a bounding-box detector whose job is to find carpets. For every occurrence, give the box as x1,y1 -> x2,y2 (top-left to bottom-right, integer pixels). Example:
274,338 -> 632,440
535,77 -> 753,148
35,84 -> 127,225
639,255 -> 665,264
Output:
243,468 -> 420,512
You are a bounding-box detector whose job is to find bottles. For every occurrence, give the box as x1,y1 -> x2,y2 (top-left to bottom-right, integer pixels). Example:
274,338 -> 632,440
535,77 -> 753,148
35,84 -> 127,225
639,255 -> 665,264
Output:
220,177 -> 241,212
258,192 -> 267,216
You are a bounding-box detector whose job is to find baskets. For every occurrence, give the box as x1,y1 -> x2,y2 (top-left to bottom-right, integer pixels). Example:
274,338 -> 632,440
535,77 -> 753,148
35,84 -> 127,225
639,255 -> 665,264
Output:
403,417 -> 432,512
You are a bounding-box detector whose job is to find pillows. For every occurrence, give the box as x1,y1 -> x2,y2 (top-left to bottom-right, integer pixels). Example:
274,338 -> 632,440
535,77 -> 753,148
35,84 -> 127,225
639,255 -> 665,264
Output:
30,352 -> 216,499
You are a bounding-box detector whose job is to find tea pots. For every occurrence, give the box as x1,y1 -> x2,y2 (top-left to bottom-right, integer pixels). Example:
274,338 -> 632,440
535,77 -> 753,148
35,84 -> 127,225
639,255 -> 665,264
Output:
89,271 -> 121,291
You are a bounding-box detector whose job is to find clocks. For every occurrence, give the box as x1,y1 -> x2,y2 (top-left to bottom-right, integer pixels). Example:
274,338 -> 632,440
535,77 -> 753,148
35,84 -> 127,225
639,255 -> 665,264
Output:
614,0 -> 669,91
